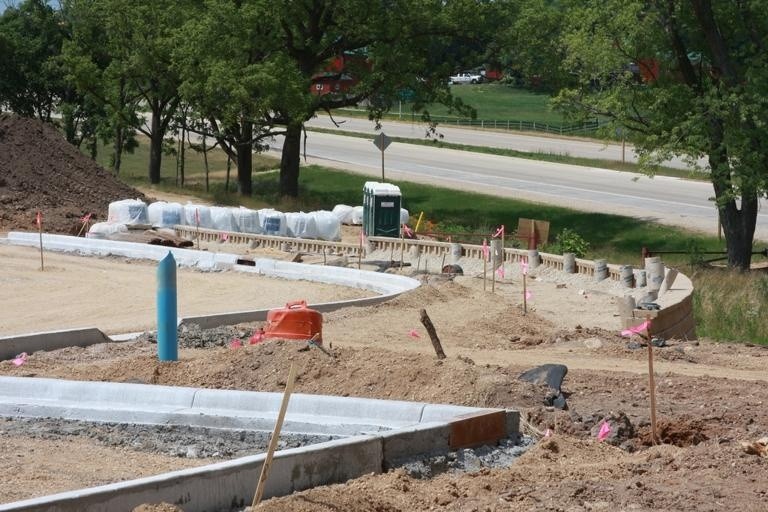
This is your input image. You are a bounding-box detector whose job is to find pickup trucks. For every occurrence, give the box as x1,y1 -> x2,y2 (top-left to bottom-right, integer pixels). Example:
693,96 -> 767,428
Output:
447,71 -> 482,85
